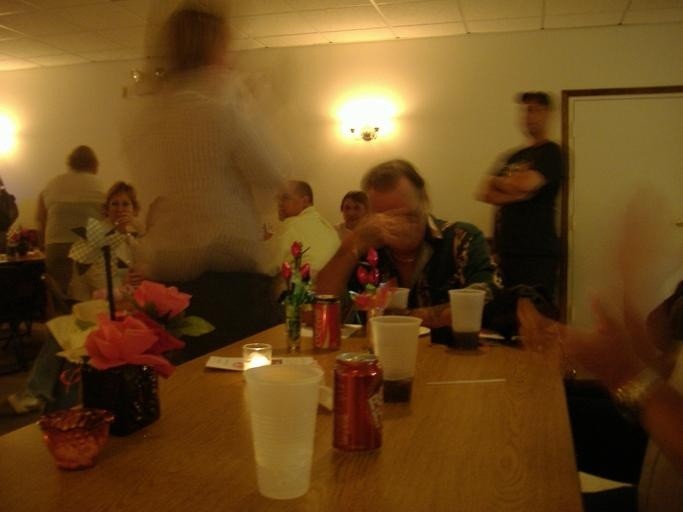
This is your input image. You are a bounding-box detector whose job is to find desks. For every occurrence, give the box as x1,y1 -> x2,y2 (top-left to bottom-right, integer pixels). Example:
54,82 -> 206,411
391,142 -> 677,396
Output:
0,314 -> 587,512
0,248 -> 47,371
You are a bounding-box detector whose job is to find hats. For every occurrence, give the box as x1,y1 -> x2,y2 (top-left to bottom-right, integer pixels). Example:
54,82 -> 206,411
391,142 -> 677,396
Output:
520,90 -> 552,108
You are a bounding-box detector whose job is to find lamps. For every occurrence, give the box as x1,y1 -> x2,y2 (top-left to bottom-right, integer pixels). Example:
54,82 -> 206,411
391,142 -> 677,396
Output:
342,121 -> 382,141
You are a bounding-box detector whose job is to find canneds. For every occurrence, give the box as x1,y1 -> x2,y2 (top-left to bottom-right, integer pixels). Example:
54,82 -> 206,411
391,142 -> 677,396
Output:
333,353 -> 383,452
313,295 -> 342,350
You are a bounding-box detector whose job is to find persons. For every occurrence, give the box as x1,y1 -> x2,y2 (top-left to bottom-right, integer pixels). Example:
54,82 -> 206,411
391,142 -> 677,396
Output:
477,90 -> 570,321
0,0 -> 366,403
297,157 -> 507,334
559,275 -> 683,511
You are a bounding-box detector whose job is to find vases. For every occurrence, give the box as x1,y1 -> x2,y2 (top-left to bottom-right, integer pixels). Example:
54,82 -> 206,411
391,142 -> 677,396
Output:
81,365 -> 161,438
284,303 -> 300,352
364,308 -> 385,351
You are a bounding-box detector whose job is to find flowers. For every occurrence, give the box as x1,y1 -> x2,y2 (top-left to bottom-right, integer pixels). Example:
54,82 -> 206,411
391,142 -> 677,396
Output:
7,276 -> 215,412
346,248 -> 393,313
274,240 -> 313,339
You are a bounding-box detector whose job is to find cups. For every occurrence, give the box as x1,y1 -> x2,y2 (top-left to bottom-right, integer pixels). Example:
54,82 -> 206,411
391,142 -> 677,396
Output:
242,363 -> 323,500
241,342 -> 270,369
369,314 -> 423,399
37,409 -> 113,472
448,288 -> 487,350
5,243 -> 16,256
384,288 -> 411,312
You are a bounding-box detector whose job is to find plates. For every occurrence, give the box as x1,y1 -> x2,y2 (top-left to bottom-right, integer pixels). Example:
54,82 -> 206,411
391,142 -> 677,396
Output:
417,326 -> 431,337
